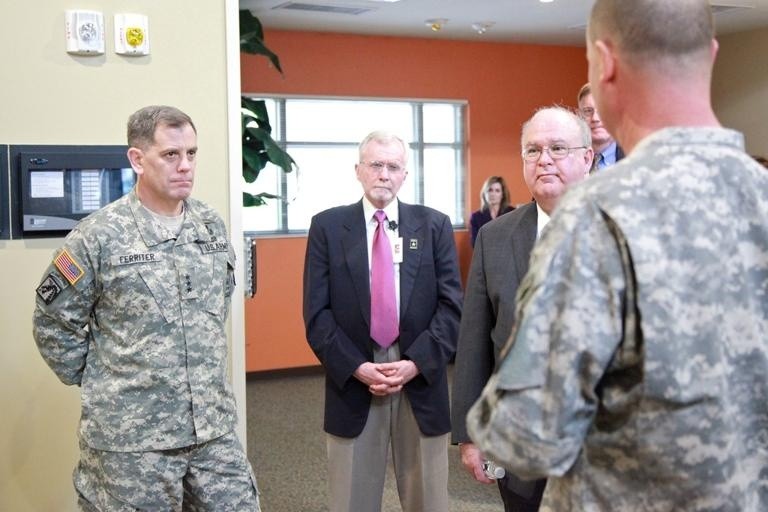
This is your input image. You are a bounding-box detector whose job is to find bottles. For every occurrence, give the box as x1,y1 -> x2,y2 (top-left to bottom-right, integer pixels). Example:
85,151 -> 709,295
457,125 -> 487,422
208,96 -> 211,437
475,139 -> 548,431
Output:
481,459 -> 506,481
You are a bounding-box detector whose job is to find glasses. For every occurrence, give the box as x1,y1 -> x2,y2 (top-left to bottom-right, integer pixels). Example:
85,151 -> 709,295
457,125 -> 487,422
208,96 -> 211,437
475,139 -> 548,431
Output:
520,143 -> 587,162
361,160 -> 400,173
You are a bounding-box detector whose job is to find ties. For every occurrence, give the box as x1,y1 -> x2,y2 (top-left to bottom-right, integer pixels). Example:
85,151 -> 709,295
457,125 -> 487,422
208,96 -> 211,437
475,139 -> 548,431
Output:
369,211 -> 400,350
589,153 -> 603,176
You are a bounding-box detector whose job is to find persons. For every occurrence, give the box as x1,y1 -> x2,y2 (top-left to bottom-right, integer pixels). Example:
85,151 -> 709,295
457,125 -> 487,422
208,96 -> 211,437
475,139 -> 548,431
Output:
448,100 -> 595,512
468,174 -> 517,247
302,127 -> 462,510
463,1 -> 767,512
31,104 -> 261,512
576,83 -> 625,176
